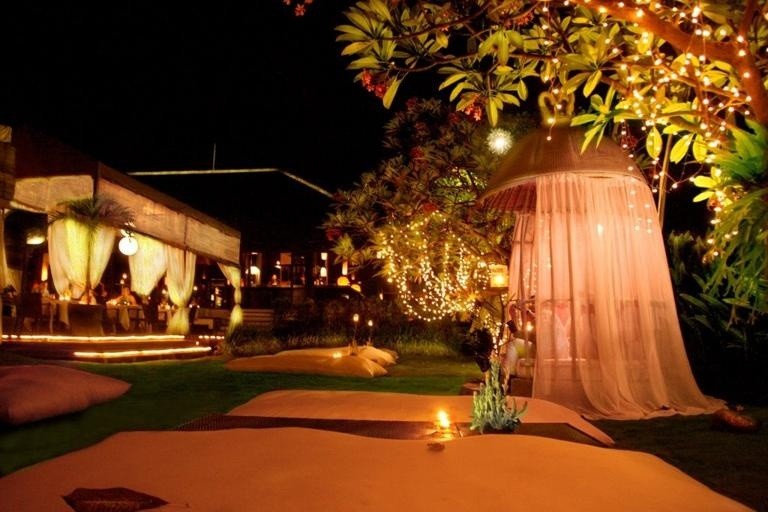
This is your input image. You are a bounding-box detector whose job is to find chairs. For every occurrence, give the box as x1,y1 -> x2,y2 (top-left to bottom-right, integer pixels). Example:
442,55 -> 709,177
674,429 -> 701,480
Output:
0,287 -> 169,336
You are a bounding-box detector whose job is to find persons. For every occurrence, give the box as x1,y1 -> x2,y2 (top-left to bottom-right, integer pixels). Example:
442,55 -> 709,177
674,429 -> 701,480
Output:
269,273 -> 279,288
33,282 -> 185,330
500,293 -> 600,370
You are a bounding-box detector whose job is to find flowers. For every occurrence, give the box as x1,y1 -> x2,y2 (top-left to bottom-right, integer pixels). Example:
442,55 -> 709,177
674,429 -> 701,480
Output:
470,349 -> 525,430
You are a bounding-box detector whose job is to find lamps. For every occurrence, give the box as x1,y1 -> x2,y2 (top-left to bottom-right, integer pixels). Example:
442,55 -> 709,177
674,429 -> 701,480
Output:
489,259 -> 510,289
119,223 -> 139,256
